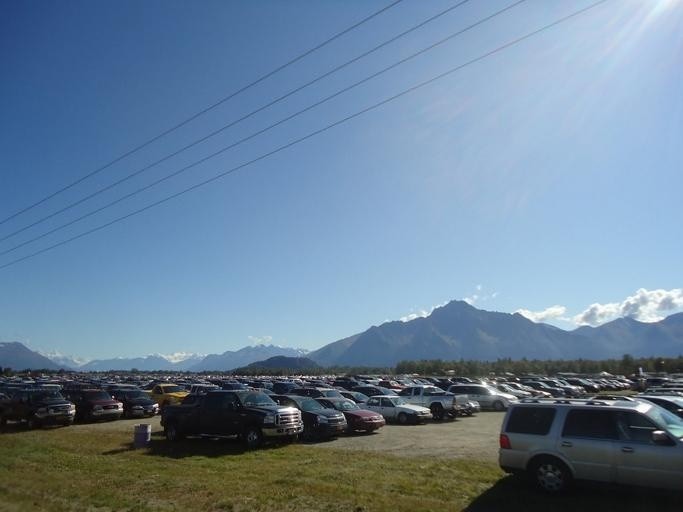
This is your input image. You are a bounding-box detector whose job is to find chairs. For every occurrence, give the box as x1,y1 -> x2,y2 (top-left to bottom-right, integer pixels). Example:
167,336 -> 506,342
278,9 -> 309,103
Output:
613,414 -> 632,441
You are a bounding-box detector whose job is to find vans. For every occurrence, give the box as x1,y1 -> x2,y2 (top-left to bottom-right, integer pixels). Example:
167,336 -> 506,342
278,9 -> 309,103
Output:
499,395 -> 681,495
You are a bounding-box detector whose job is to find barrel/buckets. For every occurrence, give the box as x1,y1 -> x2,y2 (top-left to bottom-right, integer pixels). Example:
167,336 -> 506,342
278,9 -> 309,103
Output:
133,423 -> 151,447
